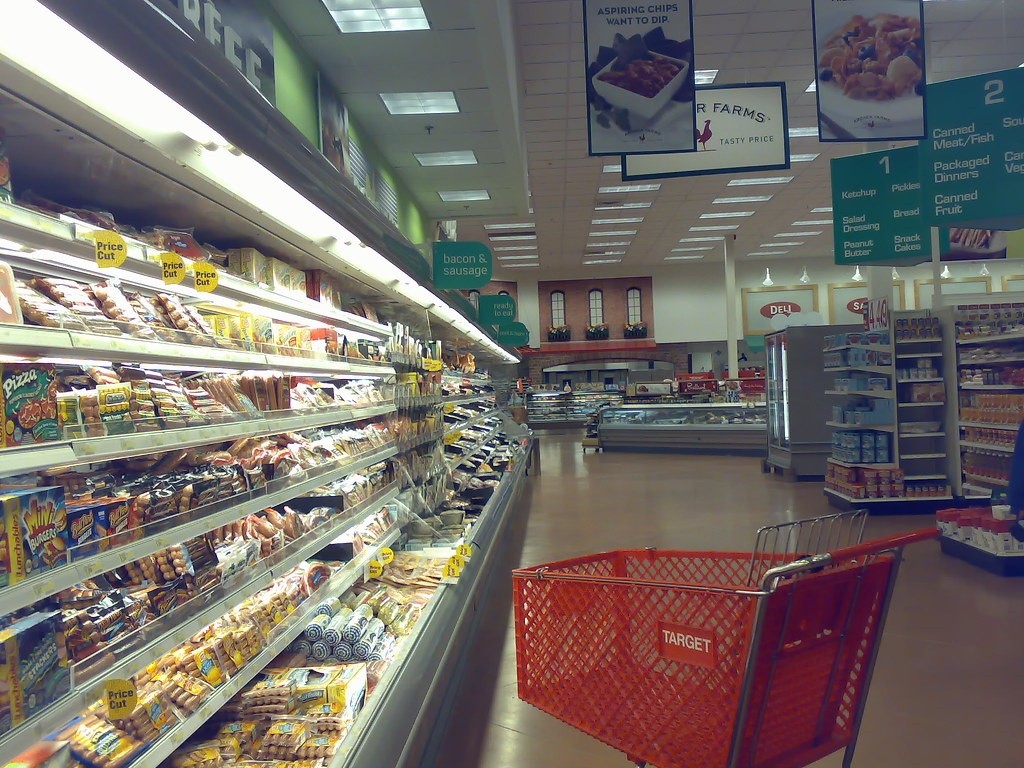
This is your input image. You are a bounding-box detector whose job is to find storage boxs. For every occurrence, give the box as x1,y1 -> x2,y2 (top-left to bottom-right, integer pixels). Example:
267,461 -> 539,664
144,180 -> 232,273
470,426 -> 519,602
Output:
0,249 -> 521,739
824,302 -> 1024,501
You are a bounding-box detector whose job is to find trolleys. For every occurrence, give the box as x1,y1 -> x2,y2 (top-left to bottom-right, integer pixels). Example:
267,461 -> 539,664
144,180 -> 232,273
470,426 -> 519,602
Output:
582,397 -> 623,452
510,506 -> 939,768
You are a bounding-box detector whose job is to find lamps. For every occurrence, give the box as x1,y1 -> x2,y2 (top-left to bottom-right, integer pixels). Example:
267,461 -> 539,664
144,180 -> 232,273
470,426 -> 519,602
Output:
762,268 -> 773,286
891,267 -> 900,280
851,265 -> 864,282
800,267 -> 811,284
979,264 -> 989,276
940,265 -> 951,278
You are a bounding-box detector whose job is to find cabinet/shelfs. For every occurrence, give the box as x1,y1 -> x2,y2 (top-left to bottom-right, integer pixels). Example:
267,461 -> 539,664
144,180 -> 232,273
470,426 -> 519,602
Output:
512,378 -> 531,397
895,337 -> 947,480
524,390 -> 626,423
822,343 -> 896,470
955,332 -> 1024,487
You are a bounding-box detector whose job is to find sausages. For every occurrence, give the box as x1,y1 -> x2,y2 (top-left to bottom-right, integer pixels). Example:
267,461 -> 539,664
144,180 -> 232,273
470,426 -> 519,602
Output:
0,195 -> 388,768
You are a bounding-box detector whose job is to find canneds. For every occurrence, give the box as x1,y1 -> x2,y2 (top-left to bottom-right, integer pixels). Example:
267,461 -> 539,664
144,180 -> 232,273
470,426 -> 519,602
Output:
963,426 -> 1018,446
902,357 -> 937,380
824,430 -> 904,499
